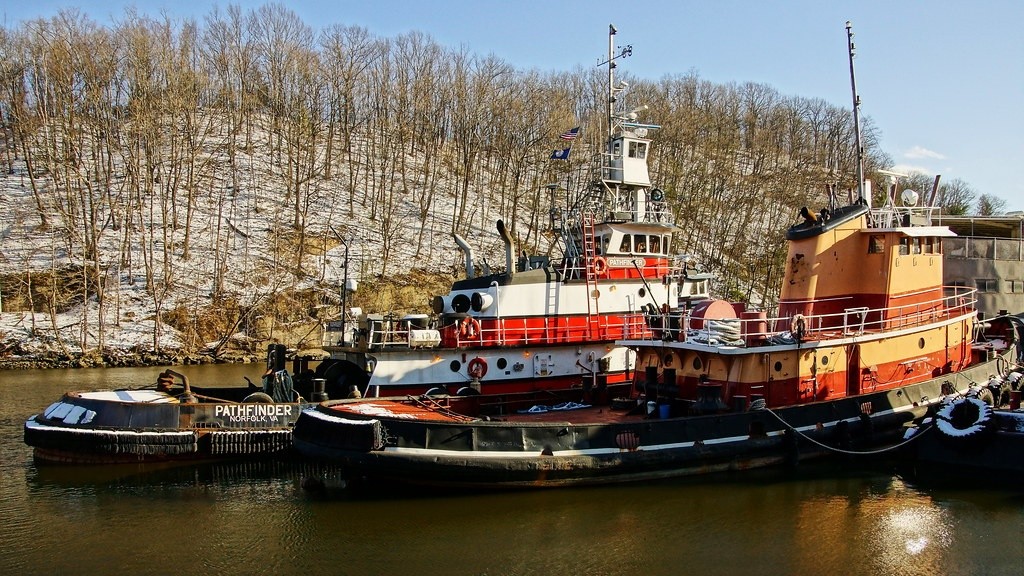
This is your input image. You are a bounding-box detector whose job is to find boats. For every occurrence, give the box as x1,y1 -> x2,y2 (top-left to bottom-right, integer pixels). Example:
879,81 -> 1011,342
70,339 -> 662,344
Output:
24,23 -> 721,469
296,24 -> 1024,501
935,366 -> 1024,459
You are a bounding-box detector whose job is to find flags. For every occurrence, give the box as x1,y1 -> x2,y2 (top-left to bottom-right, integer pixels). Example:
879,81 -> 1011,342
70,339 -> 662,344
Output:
549,148 -> 570,159
560,127 -> 579,141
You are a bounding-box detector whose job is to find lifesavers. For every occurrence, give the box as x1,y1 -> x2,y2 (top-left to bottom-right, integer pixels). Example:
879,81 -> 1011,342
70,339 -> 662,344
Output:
468,358 -> 488,379
790,314 -> 809,340
592,257 -> 607,275
461,318 -> 480,340
966,370 -> 1024,408
242,392 -> 275,404
932,397 -> 994,447
